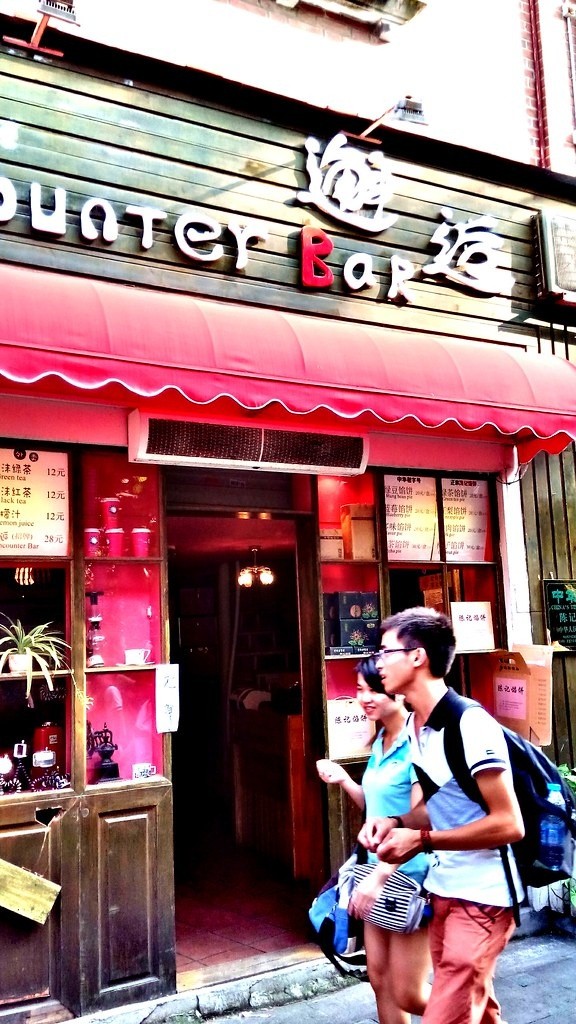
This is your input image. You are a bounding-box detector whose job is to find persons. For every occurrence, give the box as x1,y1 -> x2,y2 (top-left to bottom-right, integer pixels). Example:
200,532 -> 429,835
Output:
314,654 -> 431,1024
356,604 -> 525,1024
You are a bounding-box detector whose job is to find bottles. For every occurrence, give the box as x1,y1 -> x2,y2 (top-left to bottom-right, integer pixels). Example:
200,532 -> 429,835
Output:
540,782 -> 566,870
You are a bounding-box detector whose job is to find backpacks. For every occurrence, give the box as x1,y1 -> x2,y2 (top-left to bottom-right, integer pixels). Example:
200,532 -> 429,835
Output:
444,695 -> 576,888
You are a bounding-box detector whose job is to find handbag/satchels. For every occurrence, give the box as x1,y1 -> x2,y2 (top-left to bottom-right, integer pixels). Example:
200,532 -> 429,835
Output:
309,840 -> 370,981
353,863 -> 433,932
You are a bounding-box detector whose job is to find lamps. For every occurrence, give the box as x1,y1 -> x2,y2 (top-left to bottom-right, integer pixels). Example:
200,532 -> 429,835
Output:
341,93 -> 430,144
3,0 -> 81,58
238,546 -> 274,588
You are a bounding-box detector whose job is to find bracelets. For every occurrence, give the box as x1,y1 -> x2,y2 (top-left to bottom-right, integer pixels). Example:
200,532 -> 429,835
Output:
421,827 -> 433,855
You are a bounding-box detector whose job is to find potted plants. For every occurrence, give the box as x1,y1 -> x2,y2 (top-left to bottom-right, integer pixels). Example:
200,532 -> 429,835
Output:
0,612 -> 94,712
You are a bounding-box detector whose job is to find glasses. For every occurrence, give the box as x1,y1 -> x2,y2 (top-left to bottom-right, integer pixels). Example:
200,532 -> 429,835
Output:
378,647 -> 417,659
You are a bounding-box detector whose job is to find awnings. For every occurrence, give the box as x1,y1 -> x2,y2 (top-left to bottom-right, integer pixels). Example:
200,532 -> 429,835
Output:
0,262 -> 576,464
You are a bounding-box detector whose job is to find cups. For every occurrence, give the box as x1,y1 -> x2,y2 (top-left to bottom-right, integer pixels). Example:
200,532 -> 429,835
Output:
132,762 -> 156,780
84,528 -> 100,558
105,528 -> 125,558
124,649 -> 151,665
131,528 -> 151,558
101,497 -> 120,530
